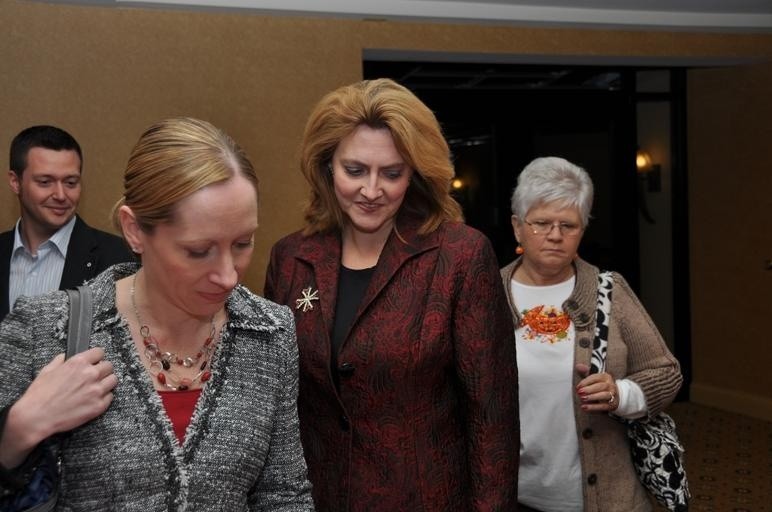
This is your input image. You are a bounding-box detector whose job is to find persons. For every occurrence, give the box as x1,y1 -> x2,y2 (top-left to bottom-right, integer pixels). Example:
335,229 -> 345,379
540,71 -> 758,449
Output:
261,77 -> 522,511
498,156 -> 686,512
0,117 -> 316,511
0,125 -> 140,320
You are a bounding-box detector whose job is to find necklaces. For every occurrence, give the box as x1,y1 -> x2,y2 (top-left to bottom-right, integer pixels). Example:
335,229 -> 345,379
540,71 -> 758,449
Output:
130,272 -> 218,393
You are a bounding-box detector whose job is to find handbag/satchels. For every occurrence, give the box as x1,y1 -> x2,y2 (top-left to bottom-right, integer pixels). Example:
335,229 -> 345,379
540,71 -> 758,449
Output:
624,410 -> 692,511
0,287 -> 97,512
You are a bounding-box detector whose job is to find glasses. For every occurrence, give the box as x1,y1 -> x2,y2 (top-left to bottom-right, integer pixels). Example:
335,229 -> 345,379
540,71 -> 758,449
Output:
523,218 -> 582,238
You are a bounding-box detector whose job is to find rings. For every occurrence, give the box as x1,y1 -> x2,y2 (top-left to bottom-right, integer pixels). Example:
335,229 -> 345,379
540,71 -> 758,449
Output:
609,391 -> 614,405
